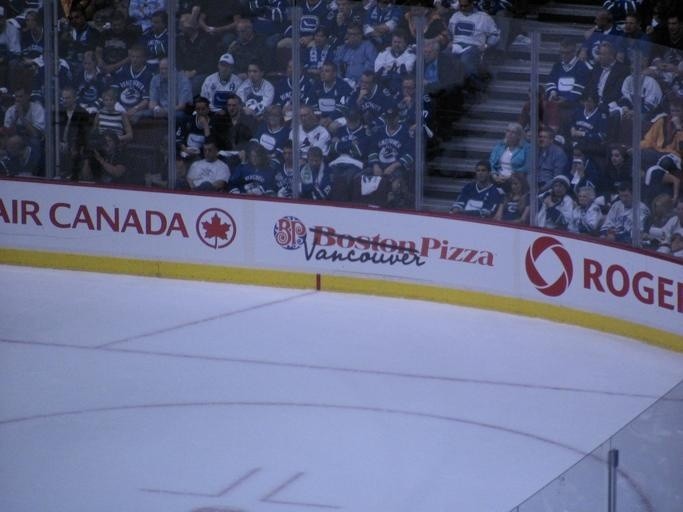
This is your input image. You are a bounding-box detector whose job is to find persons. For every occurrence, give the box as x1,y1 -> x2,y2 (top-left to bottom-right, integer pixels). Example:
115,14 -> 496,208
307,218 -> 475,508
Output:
446,1 -> 683,263
0,1 -> 452,208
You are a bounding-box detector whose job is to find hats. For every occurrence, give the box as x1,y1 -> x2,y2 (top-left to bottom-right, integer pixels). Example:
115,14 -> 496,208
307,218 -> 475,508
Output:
552,175 -> 570,192
218,54 -> 235,67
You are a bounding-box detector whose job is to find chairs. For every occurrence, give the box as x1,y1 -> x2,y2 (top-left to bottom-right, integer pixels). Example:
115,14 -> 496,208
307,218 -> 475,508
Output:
1,0 -> 681,212
